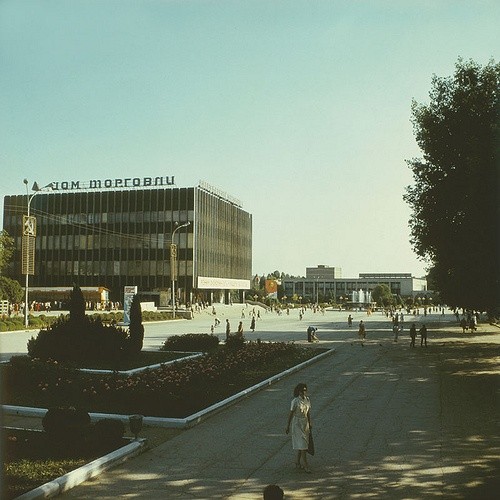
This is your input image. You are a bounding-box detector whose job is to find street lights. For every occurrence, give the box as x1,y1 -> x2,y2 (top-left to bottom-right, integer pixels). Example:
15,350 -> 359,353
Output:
21,178 -> 55,328
170,221 -> 190,319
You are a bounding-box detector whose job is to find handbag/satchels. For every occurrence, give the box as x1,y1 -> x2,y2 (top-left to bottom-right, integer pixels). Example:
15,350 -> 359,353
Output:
305,429 -> 314,454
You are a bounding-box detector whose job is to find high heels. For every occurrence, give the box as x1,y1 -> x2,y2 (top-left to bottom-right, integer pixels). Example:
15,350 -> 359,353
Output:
296,462 -> 304,468
303,464 -> 310,473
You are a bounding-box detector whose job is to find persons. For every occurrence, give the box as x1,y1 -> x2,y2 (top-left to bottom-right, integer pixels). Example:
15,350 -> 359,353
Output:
358,320 -> 365,340
453,310 -> 481,335
250,316 -> 256,332
226,319 -> 230,339
271,304 -> 290,317
381,303 -> 445,319
263,484 -> 283,500
285,383 -> 313,473
211,324 -> 214,333
307,327 -> 318,343
301,303 -> 326,316
409,324 -> 418,347
392,313 -> 404,324
238,321 -> 242,331
419,325 -> 427,346
214,319 -> 221,328
348,315 -> 353,327
393,322 -> 399,342
298,310 -> 302,320
241,307 -> 262,321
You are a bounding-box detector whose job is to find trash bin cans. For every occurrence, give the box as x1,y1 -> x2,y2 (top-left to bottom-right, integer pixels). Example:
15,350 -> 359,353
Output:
129,414 -> 144,442
